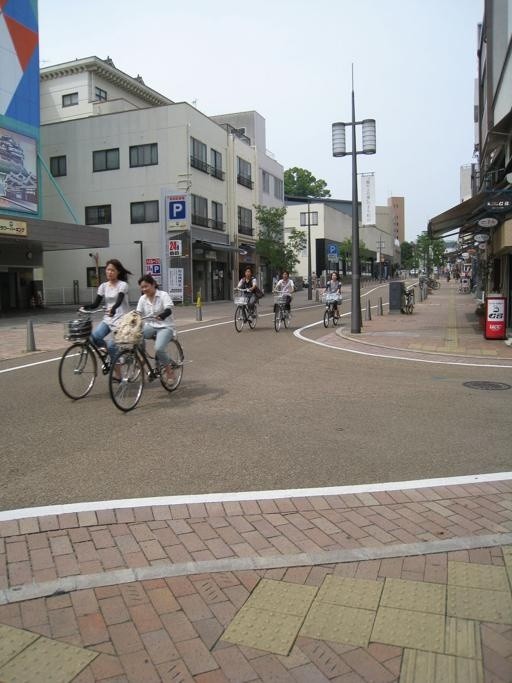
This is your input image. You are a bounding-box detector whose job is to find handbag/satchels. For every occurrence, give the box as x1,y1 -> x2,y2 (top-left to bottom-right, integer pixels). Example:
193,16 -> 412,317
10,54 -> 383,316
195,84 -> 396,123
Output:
337,293 -> 343,305
116,310 -> 143,343
70,319 -> 90,336
254,287 -> 264,298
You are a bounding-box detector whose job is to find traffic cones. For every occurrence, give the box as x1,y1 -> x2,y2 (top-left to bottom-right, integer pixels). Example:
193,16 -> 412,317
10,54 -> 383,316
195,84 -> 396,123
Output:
195,295 -> 204,320
365,297 -> 371,321
378,295 -> 384,317
22,319 -> 40,352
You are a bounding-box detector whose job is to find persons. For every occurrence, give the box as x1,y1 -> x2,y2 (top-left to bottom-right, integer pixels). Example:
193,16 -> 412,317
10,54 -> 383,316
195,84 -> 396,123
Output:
272,272 -> 295,319
235,265 -> 258,323
321,272 -> 342,318
135,274 -> 177,386
76,259 -> 132,384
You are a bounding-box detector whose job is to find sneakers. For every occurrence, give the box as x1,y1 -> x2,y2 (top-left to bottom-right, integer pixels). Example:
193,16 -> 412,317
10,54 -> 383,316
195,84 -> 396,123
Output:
166,375 -> 177,387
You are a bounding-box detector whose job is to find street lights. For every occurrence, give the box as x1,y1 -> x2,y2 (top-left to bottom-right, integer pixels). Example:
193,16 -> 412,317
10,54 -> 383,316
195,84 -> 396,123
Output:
299,200 -> 318,299
331,63 -> 376,331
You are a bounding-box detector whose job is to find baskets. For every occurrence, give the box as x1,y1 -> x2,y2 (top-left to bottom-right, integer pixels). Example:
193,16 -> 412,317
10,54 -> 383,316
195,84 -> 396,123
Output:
233,293 -> 249,305
404,288 -> 414,296
321,294 -> 336,303
63,321 -> 90,342
111,324 -> 142,349
274,296 -> 286,304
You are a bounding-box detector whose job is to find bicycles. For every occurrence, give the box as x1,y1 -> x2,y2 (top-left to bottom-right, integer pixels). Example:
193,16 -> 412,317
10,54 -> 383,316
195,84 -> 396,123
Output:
400,287 -> 416,314
233,286 -> 259,332
58,306 -> 186,411
272,290 -> 292,331
419,276 -> 441,290
319,290 -> 342,327
